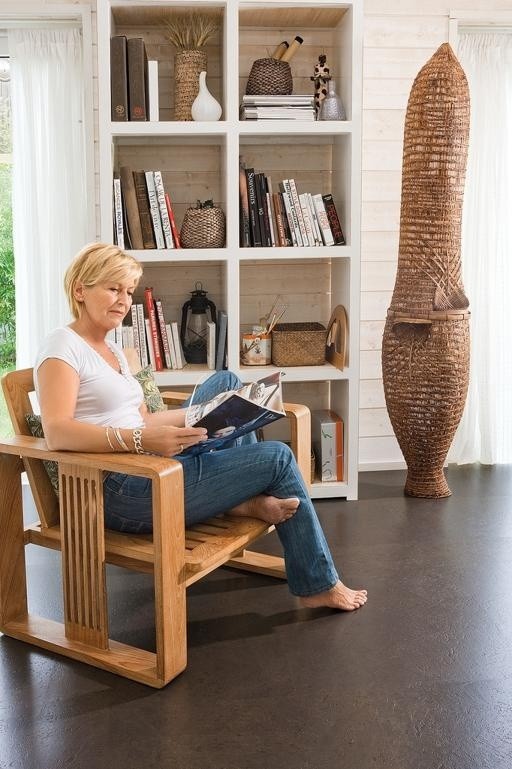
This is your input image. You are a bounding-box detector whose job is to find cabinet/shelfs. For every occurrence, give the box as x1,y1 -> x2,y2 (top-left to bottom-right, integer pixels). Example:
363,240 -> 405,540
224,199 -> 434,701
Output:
98,0 -> 364,500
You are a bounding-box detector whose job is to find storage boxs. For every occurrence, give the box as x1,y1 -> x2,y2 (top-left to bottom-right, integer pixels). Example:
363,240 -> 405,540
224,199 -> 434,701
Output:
312,411 -> 344,481
243,334 -> 271,365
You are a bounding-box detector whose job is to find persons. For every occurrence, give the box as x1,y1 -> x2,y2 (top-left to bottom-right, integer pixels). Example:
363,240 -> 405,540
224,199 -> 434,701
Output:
33,242 -> 368,610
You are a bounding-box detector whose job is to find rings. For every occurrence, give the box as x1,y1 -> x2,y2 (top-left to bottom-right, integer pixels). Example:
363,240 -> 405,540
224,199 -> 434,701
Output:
180,445 -> 183,453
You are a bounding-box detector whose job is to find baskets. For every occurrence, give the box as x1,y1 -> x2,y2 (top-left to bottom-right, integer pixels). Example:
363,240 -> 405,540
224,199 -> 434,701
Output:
180,207 -> 225,248
174,50 -> 207,121
246,58 -> 293,95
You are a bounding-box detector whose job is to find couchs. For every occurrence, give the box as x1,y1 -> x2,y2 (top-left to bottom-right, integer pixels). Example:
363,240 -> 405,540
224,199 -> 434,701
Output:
0,366 -> 309,688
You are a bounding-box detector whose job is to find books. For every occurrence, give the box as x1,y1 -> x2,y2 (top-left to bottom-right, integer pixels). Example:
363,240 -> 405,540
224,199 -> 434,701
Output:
217,309 -> 227,370
205,319 -> 215,370
242,95 -> 317,123
175,371 -> 287,458
115,286 -> 187,371
239,155 -> 347,247
111,35 -> 160,122
114,166 -> 181,249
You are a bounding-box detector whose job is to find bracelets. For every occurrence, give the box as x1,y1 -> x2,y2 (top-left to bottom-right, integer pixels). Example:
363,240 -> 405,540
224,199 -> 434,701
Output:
106,425 -> 130,452
133,429 -> 145,454
